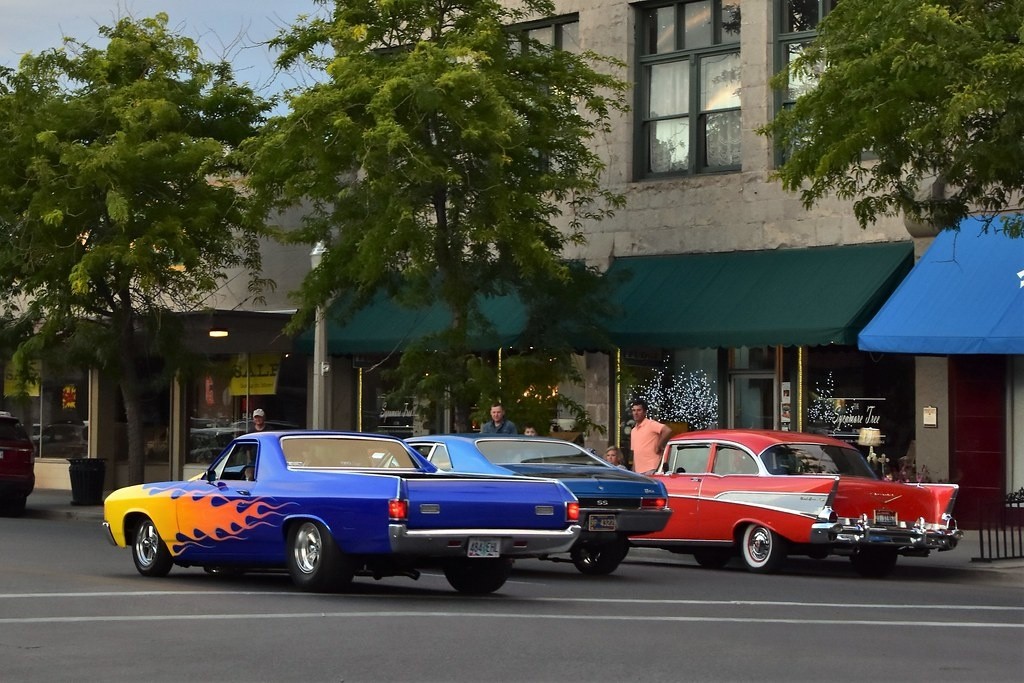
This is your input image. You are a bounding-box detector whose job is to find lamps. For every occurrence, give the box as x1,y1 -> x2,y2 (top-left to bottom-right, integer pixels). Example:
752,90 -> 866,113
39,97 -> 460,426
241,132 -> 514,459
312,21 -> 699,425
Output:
858,428 -> 882,468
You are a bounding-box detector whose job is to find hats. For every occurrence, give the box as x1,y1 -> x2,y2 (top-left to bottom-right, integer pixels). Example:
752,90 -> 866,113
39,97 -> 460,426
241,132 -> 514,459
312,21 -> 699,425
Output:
253,409 -> 265,417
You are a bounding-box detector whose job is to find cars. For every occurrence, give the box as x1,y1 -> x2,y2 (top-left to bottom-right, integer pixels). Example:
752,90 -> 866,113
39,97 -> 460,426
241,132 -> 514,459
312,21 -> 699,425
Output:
374,437 -> 672,578
28,420 -> 89,458
627,430 -> 964,574
190,416 -> 300,465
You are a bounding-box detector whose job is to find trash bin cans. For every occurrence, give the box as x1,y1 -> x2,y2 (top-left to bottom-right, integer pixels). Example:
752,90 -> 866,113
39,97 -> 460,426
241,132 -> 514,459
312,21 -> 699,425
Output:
66,457 -> 109,507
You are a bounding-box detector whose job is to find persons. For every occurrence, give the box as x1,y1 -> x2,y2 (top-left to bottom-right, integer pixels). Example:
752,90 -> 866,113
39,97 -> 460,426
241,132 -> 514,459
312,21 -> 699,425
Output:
605,446 -> 628,470
522,423 -> 539,437
247,409 -> 274,432
481,402 -> 517,435
245,468 -> 255,483
630,401 -> 672,475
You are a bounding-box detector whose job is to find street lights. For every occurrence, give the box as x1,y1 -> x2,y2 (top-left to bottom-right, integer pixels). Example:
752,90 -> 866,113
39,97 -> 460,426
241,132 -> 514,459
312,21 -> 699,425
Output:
310,238 -> 332,433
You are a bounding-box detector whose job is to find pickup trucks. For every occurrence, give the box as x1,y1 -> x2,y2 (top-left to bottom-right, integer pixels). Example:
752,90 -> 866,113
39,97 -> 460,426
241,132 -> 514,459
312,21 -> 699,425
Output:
101,431 -> 582,597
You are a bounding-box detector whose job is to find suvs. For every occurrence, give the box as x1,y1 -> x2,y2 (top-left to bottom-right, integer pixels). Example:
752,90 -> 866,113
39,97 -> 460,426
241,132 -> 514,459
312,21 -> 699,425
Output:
0,410 -> 35,518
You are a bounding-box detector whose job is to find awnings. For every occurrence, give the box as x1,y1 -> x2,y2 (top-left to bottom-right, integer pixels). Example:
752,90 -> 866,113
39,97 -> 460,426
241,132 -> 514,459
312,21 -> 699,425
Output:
857,214 -> 1024,355
289,240 -> 912,354
